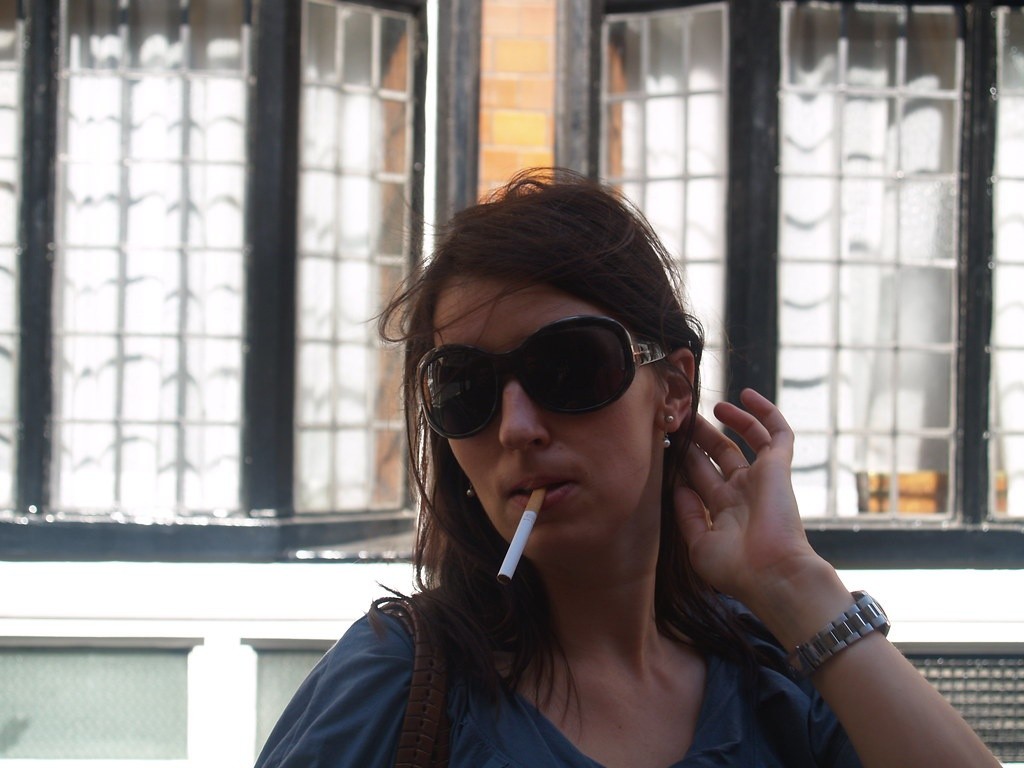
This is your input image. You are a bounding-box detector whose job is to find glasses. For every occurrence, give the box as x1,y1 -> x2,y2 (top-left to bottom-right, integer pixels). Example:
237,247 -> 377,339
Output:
417,315 -> 692,440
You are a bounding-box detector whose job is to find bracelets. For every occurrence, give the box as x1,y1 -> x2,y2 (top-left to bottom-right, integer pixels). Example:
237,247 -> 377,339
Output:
791,588 -> 894,676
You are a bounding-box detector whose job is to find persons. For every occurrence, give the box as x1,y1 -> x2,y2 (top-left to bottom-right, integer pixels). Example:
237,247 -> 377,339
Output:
251,177 -> 1004,768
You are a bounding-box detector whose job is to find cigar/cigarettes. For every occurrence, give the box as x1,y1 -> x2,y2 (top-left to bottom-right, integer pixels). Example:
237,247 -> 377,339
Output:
497,488 -> 548,588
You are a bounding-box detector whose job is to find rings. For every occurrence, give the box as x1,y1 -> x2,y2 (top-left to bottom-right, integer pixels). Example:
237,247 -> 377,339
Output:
725,464 -> 751,482
